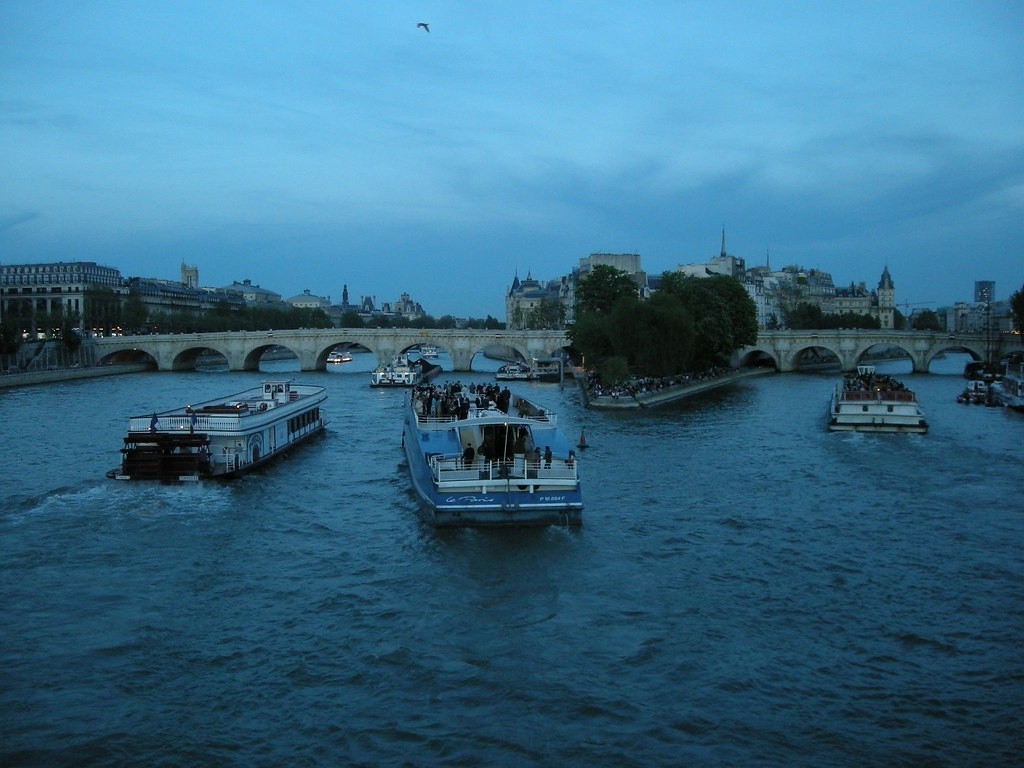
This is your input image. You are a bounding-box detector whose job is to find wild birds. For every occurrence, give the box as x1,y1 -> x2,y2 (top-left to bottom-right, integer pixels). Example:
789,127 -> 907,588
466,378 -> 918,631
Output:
416,22 -> 431,32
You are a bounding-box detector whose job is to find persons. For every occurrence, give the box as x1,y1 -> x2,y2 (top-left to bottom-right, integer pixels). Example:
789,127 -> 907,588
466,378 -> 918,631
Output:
844,371 -> 910,398
586,366 -> 739,401
231,443 -> 242,470
412,380 -> 553,476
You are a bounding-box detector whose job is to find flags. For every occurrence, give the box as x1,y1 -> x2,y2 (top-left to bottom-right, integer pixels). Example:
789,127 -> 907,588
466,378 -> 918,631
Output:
190,412 -> 196,434
151,413 -> 158,433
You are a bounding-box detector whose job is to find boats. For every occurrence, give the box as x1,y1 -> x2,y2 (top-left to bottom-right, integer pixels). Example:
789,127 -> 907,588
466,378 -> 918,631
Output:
536,362 -> 560,380
327,350 -> 354,362
987,362 -> 1024,409
420,345 -> 438,359
106,378 -> 332,480
369,352 -> 442,388
400,385 -> 586,528
496,361 -> 531,380
824,365 -> 931,435
967,380 -> 987,399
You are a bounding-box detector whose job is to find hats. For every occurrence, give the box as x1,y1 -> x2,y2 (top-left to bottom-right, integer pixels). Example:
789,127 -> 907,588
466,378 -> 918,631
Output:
545,446 -> 550,450
467,443 -> 471,446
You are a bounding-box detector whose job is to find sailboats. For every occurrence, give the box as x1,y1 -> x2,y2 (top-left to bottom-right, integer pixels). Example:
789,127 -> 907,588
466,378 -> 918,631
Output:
970,294 -> 1006,382
